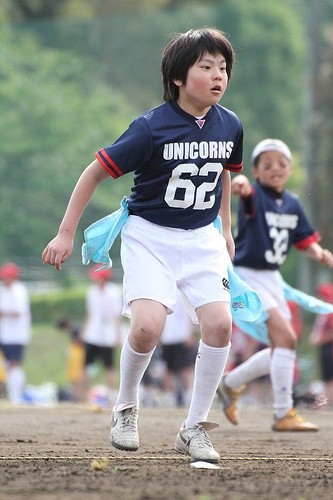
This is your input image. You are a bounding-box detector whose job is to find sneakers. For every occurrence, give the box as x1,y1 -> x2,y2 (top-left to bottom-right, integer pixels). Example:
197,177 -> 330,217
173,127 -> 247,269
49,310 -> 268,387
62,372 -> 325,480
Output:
110,405 -> 140,451
272,408 -> 318,432
216,373 -> 246,426
173,420 -> 222,462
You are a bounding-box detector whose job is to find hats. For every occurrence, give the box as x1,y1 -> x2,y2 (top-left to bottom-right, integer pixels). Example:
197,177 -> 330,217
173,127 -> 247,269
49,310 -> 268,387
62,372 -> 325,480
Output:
251,138 -> 292,164
0,262 -> 20,279
88,265 -> 110,280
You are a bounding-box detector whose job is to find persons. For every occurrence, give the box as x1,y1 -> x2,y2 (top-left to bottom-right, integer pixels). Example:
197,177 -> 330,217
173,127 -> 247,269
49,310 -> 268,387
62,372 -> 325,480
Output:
0,260 -> 32,405
41,25 -> 244,460
215,138 -> 333,431
80,262 -> 123,409
310,271 -> 333,413
161,297 -> 196,408
